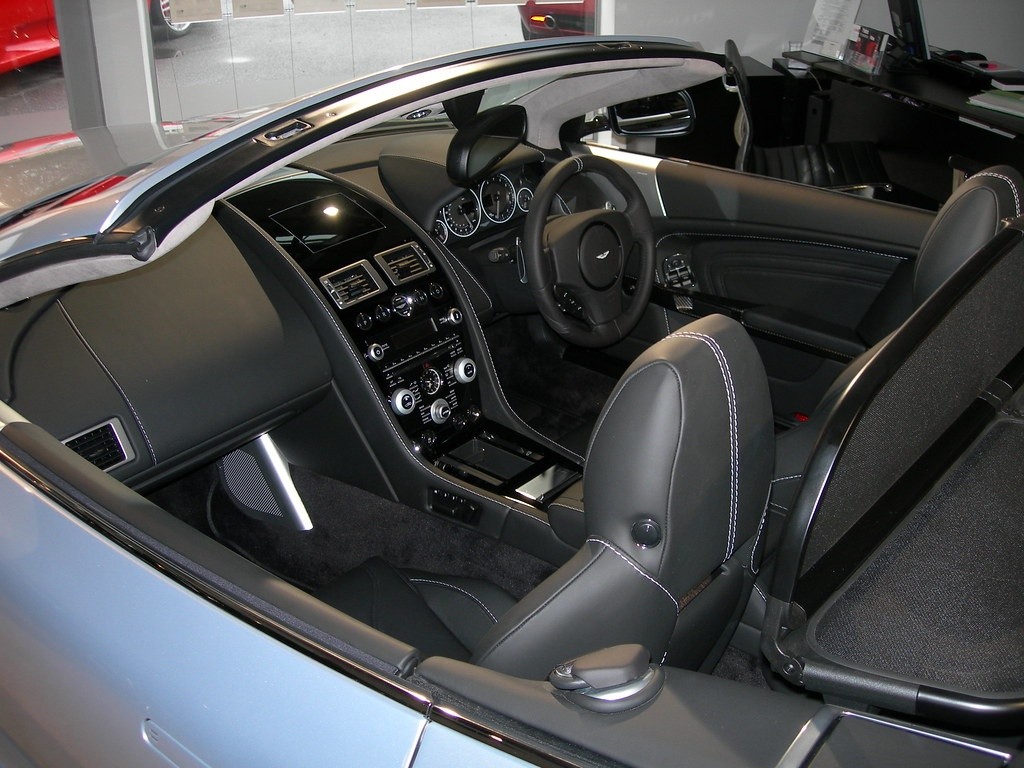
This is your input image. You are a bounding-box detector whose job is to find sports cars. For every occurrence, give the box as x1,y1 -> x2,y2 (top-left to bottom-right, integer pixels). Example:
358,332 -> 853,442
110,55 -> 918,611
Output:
517,1 -> 599,39
2,30 -> 1023,768
1,0 -> 190,74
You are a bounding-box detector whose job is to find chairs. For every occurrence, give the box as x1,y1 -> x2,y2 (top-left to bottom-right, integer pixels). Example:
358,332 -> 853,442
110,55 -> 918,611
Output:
750,163 -> 1023,579
317,305 -> 772,680
